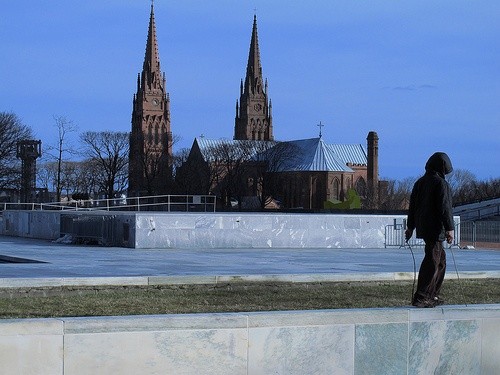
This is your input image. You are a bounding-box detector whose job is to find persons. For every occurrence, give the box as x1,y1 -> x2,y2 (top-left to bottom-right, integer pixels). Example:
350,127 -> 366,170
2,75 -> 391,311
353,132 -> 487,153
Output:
404,151 -> 454,310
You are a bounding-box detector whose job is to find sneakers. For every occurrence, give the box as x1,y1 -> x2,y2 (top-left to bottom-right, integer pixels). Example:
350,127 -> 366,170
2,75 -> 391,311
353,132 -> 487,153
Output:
432,291 -> 446,306
411,294 -> 436,309
